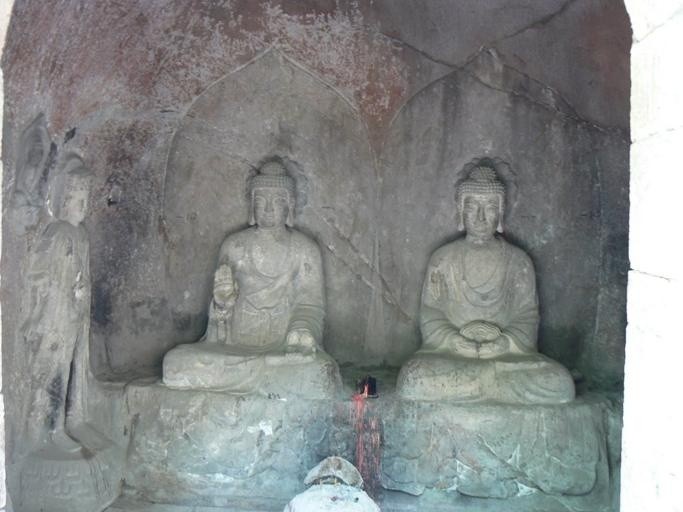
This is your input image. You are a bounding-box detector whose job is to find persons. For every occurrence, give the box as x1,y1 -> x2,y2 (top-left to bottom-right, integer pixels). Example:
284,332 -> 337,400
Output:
162,162 -> 345,401
395,166 -> 576,405
23,154 -> 95,452
